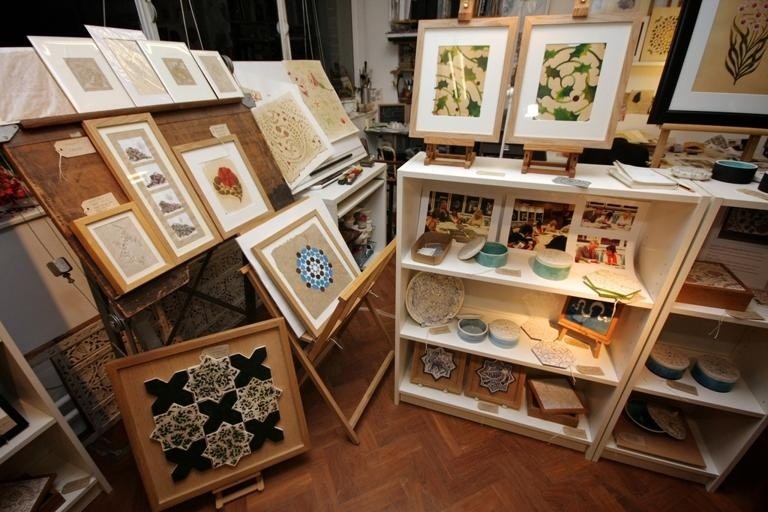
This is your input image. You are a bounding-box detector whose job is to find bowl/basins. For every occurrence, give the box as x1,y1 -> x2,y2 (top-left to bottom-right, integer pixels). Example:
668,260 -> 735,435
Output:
712,161 -> 759,184
456,317 -> 488,344
475,241 -> 508,268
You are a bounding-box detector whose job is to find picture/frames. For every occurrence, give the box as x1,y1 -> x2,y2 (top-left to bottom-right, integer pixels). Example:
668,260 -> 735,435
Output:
741,134 -> 767,163
408,16 -> 520,143
646,0 -> 768,129
70,201 -> 177,296
251,209 -> 359,339
557,296 -> 625,347
172,134 -> 274,241
409,342 -> 467,396
504,12 -> 644,149
463,355 -> 527,410
82,112 -> 224,266
106,316 -> 313,511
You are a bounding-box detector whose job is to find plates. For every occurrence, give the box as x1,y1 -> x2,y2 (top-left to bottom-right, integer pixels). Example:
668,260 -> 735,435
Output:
624,400 -> 665,435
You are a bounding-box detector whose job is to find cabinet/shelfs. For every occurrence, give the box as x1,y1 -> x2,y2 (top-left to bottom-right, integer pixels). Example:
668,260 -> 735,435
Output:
395,151 -> 709,461
0,322 -> 112,512
595,169 -> 768,495
296,163 -> 389,271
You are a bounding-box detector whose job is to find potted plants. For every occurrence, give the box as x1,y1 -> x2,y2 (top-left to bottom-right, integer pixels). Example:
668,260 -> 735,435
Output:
338,86 -> 357,114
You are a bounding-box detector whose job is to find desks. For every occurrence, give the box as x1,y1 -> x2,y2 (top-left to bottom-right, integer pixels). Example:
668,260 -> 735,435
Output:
363,125 -> 407,159
